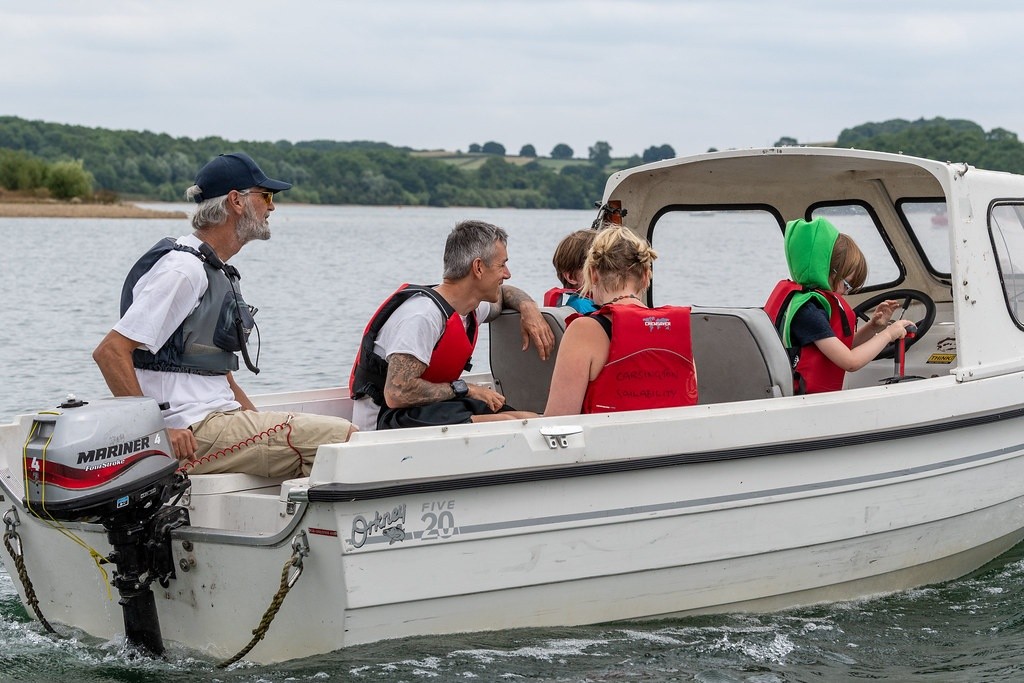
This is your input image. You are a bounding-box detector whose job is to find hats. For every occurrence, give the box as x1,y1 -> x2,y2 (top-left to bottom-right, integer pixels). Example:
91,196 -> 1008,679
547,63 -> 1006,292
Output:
193,154 -> 292,204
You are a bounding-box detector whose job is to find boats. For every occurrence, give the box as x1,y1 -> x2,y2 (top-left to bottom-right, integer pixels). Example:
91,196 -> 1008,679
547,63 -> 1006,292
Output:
2,146 -> 1023,667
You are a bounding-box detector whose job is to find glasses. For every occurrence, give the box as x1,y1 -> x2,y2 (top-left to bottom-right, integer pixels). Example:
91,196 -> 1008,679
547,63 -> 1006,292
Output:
832,267 -> 853,295
250,189 -> 273,204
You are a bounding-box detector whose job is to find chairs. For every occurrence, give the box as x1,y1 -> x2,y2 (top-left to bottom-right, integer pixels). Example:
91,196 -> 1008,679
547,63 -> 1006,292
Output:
488,305 -> 580,414
689,305 -> 794,404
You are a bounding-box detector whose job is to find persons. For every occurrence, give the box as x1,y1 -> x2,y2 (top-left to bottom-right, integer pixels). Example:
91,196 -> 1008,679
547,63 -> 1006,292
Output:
91,152 -> 358,477
544,230 -> 600,313
350,221 -> 555,432
763,217 -> 918,396
544,224 -> 698,417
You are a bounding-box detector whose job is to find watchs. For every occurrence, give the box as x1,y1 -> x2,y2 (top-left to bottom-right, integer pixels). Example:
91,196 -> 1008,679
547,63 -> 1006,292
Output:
450,379 -> 468,398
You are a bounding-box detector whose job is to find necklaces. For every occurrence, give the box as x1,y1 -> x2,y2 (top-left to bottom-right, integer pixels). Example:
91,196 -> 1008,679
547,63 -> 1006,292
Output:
607,294 -> 640,303
